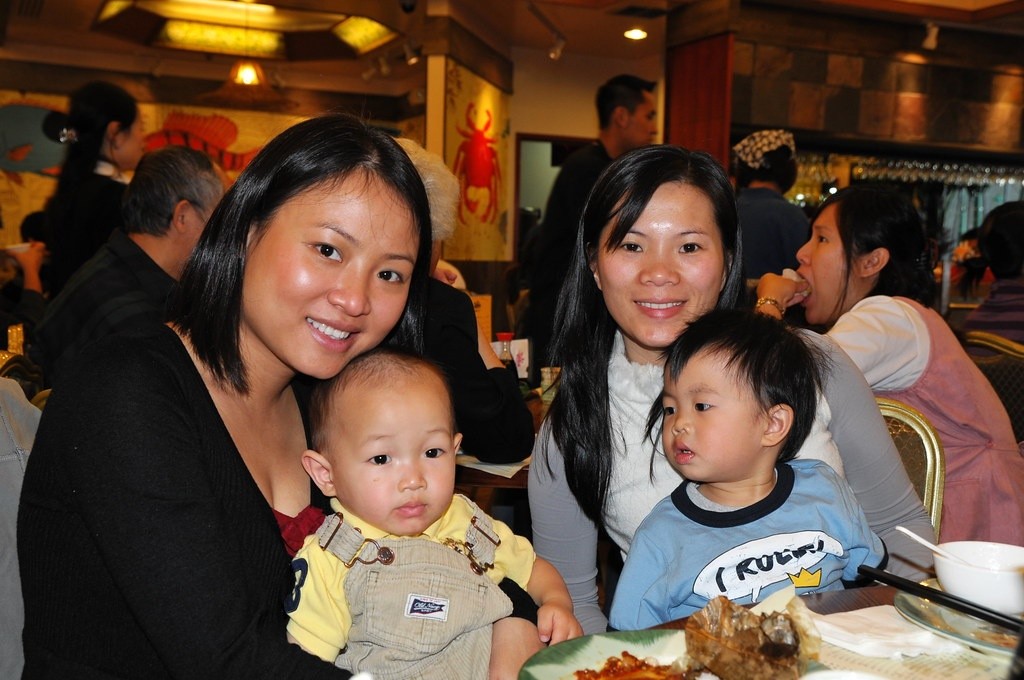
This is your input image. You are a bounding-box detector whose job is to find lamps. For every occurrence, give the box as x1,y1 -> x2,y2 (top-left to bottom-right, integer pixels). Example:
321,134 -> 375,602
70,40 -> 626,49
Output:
922,23 -> 940,49
547,37 -> 566,59
402,41 -> 419,64
200,4 -> 299,108
378,58 -> 392,74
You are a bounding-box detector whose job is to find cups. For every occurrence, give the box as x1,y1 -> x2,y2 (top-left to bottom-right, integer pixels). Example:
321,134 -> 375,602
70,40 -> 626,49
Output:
540,366 -> 562,404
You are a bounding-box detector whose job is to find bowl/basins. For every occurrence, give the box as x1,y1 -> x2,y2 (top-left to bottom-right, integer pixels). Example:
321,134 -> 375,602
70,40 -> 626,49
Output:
932,539 -> 1024,615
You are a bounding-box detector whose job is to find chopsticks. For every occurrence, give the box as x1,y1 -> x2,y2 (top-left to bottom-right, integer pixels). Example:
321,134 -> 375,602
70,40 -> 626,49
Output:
857,564 -> 1024,631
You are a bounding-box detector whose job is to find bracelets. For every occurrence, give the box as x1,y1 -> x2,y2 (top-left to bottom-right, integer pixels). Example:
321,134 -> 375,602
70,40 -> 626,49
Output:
755,297 -> 785,318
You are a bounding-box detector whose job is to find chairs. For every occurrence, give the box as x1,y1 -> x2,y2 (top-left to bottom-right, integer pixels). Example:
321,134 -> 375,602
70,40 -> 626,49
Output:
959,330 -> 1024,442
877,397 -> 946,547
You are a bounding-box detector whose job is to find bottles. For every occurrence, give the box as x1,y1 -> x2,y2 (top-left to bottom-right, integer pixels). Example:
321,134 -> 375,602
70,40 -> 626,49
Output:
497,332 -> 519,386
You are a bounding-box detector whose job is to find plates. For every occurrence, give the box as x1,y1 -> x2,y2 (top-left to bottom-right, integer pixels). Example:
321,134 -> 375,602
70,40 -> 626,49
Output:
893,577 -> 1016,657
518,628 -> 828,680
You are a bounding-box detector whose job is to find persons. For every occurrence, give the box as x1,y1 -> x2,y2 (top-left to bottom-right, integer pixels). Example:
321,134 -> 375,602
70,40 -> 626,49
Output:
733,133 -> 816,328
286,349 -> 584,680
756,181 -> 1024,549
16,114 -> 547,680
397,137 -> 535,463
608,307 -> 889,629
0,81 -> 224,390
960,201 -> 1024,442
516,74 -> 658,387
527,144 -> 937,636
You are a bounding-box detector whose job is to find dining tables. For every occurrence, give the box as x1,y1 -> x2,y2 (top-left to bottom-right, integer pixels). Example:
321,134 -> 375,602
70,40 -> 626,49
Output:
657,584 -> 1024,680
447,391 -> 543,516
